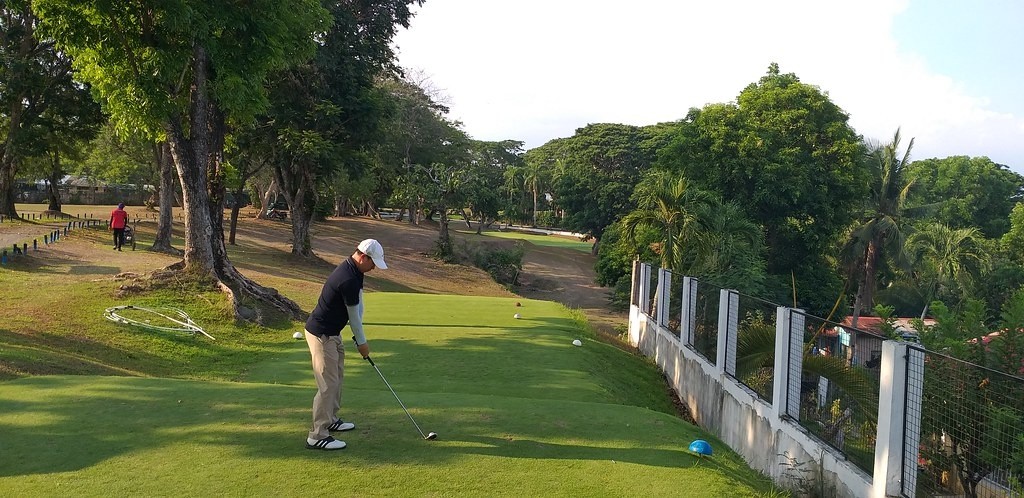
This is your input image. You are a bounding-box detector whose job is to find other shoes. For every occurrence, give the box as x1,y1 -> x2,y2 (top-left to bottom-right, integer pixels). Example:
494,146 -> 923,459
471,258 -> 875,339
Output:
113,246 -> 117,250
118,248 -> 122,251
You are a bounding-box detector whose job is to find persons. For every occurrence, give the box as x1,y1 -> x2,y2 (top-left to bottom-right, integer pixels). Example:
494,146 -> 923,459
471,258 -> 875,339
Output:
108,203 -> 128,251
303,239 -> 388,450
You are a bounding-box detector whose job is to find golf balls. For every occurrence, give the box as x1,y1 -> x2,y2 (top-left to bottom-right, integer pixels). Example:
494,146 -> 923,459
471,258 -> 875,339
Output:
429,432 -> 435,436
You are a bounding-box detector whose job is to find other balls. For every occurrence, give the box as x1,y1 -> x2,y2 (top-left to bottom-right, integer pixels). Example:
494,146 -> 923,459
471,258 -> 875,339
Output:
573,339 -> 582,346
514,314 -> 521,318
515,301 -> 521,306
292,332 -> 303,339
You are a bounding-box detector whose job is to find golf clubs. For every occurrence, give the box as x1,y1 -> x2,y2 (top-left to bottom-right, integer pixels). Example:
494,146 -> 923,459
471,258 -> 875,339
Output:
352,335 -> 438,441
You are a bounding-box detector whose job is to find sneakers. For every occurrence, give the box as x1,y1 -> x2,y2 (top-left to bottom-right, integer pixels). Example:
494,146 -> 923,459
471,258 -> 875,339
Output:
305,435 -> 346,450
327,418 -> 355,432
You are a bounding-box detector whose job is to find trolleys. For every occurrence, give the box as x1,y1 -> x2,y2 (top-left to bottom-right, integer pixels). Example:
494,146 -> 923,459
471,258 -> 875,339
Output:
123,225 -> 137,252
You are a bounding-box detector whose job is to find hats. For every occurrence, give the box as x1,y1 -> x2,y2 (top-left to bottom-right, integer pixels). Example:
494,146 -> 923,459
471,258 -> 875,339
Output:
358,239 -> 388,270
119,203 -> 124,208
